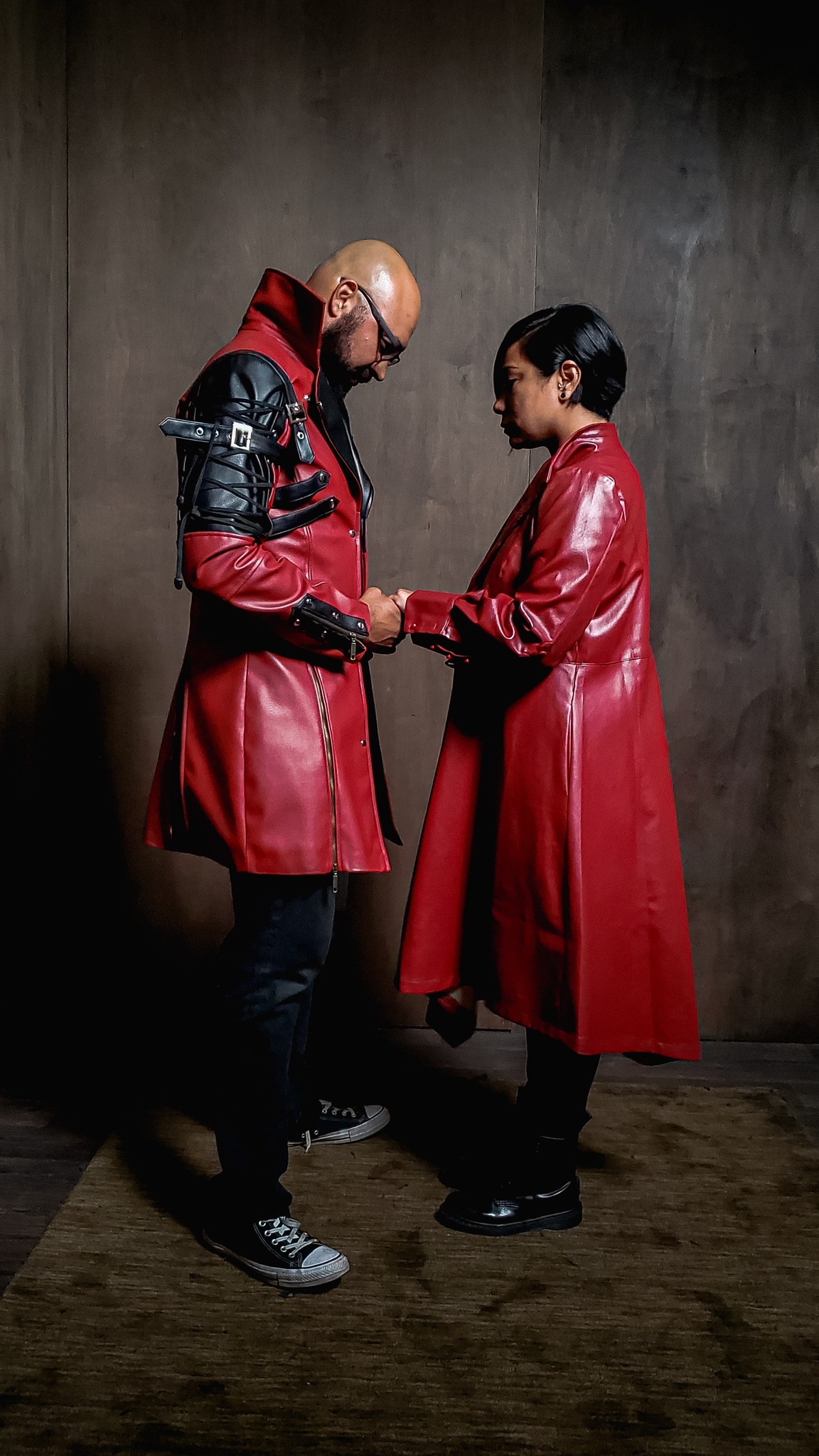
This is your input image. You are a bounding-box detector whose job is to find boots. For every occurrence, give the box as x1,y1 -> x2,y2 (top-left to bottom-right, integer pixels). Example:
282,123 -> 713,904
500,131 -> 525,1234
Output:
435,1087 -> 592,1236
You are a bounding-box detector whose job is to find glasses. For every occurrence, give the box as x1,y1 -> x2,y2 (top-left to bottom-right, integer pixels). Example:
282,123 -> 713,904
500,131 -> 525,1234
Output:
339,277 -> 400,366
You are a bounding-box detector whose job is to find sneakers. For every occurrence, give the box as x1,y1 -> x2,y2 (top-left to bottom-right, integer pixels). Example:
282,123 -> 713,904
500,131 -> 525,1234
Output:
202,1215 -> 350,1289
288,1099 -> 390,1154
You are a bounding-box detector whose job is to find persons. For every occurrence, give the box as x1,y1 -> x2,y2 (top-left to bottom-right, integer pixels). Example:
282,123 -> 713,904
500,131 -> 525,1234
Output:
378,302 -> 705,1238
134,239 -> 425,1297
